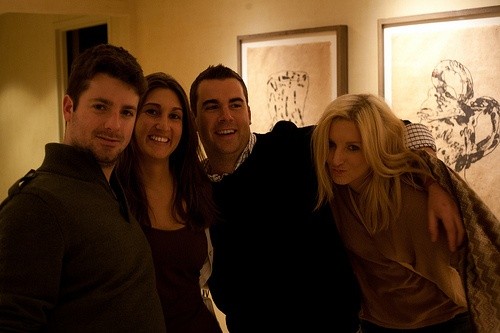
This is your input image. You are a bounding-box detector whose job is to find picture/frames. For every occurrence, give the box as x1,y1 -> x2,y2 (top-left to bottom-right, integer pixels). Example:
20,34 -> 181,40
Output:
377,5 -> 500,221
235,24 -> 348,134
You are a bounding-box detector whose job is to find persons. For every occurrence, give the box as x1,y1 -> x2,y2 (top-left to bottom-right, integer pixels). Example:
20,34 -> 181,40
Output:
189,64 -> 465,333
110,72 -> 223,333
1,43 -> 166,333
310,93 -> 500,333
